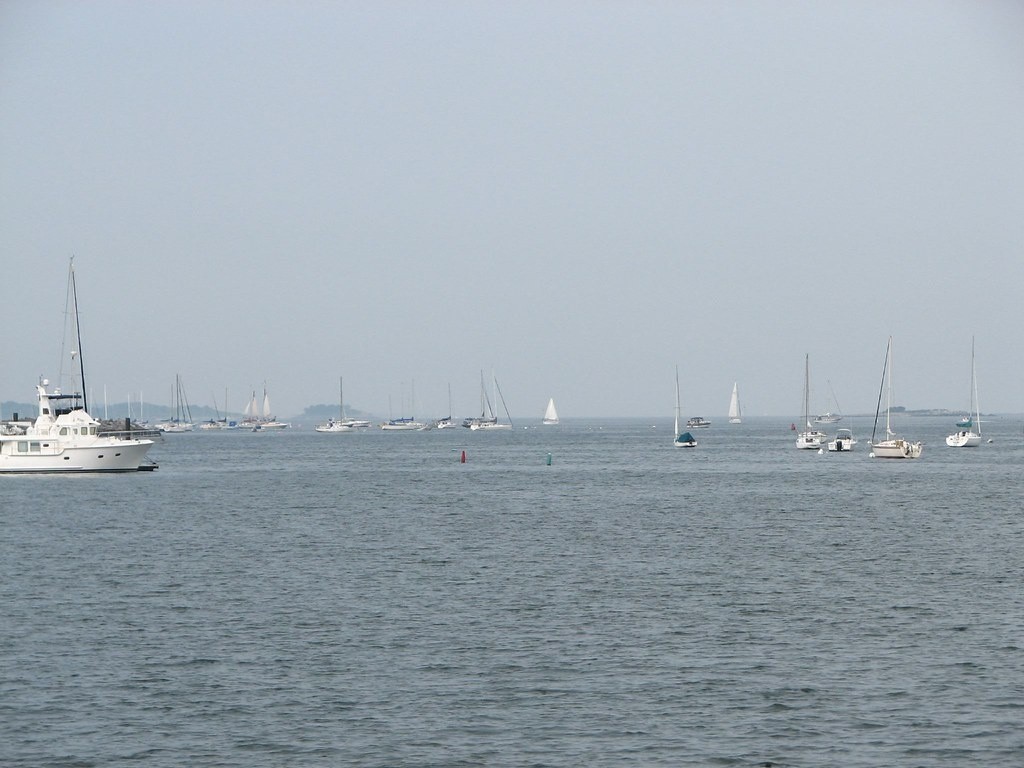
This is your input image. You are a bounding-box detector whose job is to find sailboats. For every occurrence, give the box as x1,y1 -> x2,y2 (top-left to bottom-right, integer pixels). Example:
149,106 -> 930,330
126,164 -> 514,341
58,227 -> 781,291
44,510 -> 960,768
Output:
154,369 -> 287,433
542,398 -> 560,425
673,365 -> 698,447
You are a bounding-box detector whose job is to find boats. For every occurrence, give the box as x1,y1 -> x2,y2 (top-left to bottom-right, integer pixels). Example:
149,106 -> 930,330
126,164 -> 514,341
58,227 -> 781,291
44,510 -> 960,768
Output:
438,381 -> 457,429
728,381 -> 742,424
946,336 -> 983,448
0,254 -> 166,473
828,419 -> 858,451
790,351 -> 827,450
377,378 -> 428,431
814,378 -> 844,424
687,415 -> 711,428
316,375 -> 372,432
956,418 -> 973,427
461,370 -> 514,431
867,336 -> 922,459
